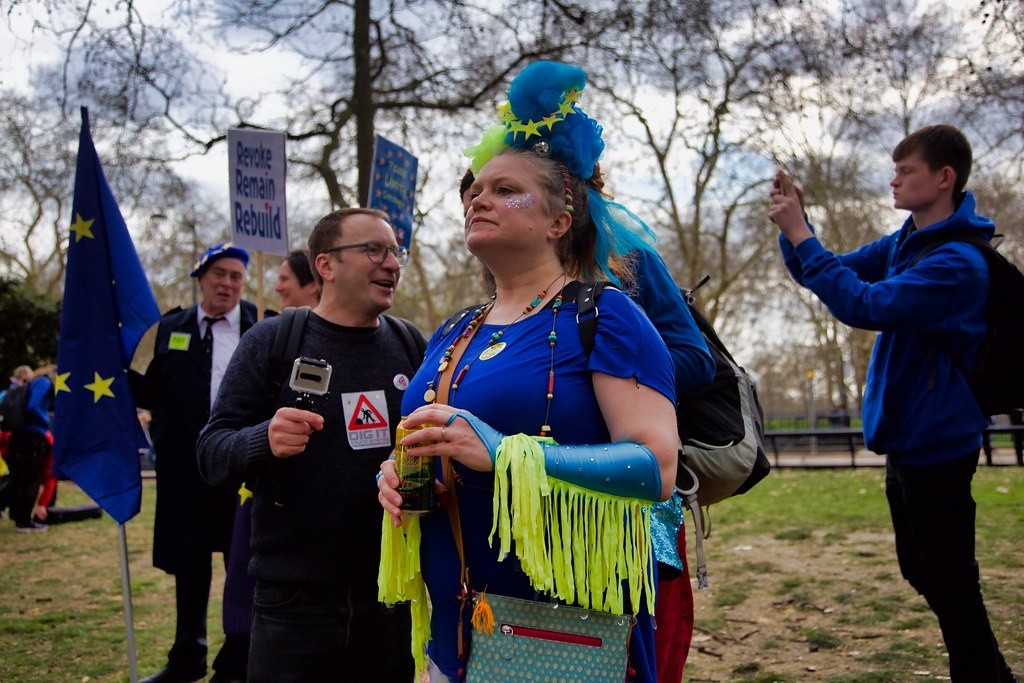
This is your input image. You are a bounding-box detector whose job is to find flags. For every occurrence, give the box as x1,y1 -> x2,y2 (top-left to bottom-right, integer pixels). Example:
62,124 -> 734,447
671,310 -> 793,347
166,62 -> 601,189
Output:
53,120 -> 162,523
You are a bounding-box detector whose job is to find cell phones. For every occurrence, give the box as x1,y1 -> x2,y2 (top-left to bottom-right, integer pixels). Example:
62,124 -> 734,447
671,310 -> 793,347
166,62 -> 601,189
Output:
778,176 -> 785,195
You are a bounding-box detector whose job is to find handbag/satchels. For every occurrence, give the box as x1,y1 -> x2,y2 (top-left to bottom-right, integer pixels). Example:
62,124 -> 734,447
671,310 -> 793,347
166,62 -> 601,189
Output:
454,583 -> 636,683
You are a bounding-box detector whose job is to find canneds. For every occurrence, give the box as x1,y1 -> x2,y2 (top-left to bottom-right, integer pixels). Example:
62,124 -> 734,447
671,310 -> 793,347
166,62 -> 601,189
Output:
392,415 -> 435,513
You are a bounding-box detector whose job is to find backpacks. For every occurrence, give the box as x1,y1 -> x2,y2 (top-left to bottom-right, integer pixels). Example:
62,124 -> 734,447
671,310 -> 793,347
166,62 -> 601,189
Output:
646,267 -> 774,508
913,236 -> 1024,415
1,374 -> 56,433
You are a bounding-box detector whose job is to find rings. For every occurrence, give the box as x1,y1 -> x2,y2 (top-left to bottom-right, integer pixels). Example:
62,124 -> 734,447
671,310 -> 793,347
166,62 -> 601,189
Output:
376,473 -> 382,483
441,427 -> 446,442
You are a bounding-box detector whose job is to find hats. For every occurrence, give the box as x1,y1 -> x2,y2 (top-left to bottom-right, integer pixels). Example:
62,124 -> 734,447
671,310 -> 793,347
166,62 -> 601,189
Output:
190,240 -> 250,278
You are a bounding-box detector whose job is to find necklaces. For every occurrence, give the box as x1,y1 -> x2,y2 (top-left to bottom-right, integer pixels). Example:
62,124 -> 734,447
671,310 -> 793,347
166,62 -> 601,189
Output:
424,273 -> 567,512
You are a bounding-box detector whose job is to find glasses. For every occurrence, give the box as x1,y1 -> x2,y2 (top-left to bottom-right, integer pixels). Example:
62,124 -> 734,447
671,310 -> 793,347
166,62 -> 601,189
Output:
325,240 -> 409,268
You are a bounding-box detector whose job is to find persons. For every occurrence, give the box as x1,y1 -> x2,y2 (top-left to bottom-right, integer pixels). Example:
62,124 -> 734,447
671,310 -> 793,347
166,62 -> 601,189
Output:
144,209 -> 434,683
0,365 -> 58,530
377,60 -> 718,683
770,124 -> 1016,683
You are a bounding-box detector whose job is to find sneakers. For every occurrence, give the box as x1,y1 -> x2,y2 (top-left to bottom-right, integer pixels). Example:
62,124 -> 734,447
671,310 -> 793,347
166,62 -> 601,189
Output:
138,651 -> 209,683
15,520 -> 49,534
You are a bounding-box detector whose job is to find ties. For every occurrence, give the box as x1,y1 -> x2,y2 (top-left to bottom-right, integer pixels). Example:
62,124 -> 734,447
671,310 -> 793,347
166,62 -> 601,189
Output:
197,316 -> 227,423
